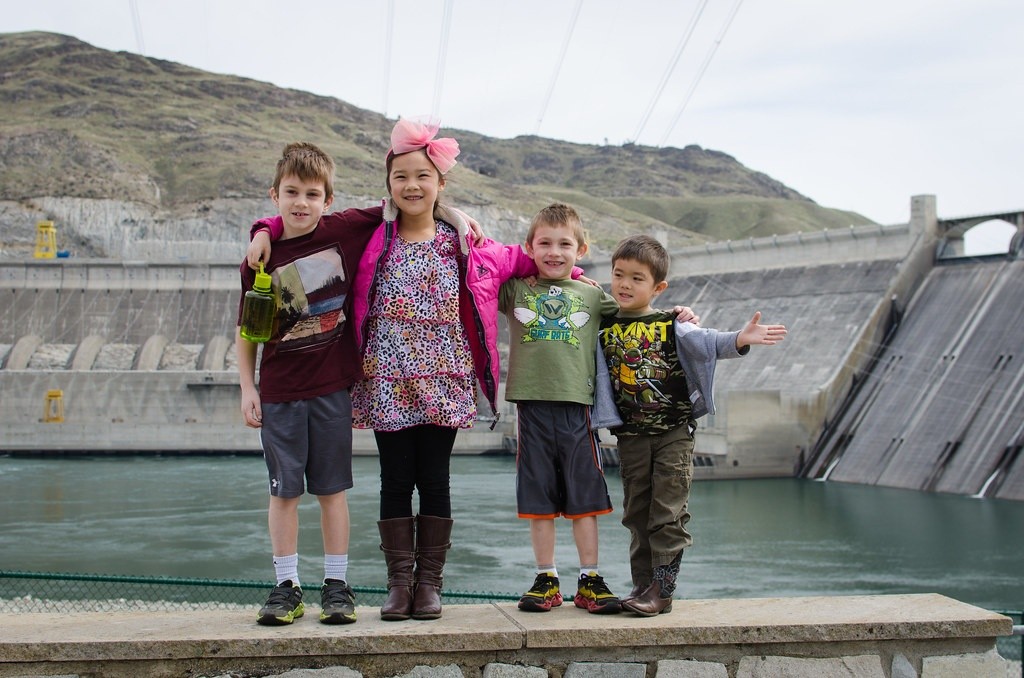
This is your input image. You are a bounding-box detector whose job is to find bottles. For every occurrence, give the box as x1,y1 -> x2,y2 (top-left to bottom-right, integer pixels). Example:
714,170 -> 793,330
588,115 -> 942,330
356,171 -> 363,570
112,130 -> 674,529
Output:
46,389 -> 64,423
240,261 -> 275,342
34,221 -> 56,258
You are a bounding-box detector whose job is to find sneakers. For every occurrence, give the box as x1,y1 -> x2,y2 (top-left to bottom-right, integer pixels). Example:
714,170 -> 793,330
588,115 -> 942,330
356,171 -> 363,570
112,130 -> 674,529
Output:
256,580 -> 304,625
574,570 -> 622,613
518,571 -> 563,611
321,578 -> 356,624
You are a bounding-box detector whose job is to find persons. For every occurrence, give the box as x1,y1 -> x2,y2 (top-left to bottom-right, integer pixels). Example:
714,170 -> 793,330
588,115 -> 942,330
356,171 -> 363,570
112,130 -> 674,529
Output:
244,117 -> 605,619
524,236 -> 787,617
497,203 -> 699,613
235,141 -> 487,628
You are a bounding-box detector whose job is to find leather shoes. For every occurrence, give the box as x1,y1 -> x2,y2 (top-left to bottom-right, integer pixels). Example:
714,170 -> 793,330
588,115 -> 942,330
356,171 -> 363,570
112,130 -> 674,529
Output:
622,579 -> 672,616
619,584 -> 650,612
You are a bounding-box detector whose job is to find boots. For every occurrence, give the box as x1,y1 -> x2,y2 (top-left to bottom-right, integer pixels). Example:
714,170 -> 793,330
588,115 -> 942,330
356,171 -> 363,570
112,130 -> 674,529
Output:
412,514 -> 453,618
377,516 -> 415,619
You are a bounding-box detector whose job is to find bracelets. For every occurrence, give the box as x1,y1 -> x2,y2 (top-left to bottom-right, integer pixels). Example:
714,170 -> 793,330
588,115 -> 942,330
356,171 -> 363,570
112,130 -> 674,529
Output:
253,228 -> 272,236
